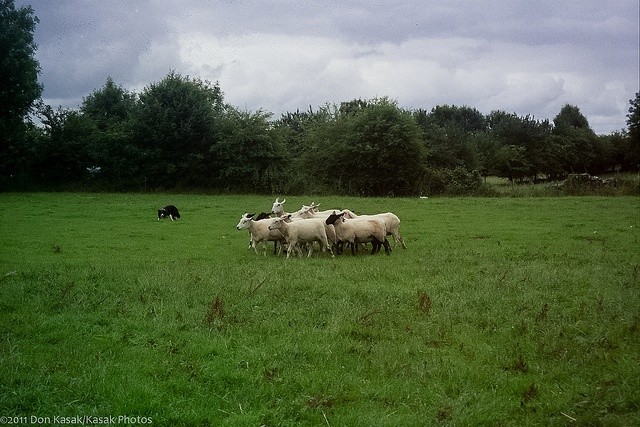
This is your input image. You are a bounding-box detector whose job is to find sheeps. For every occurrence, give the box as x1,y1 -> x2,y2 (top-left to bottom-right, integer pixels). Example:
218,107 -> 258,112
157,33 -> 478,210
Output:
236,198 -> 408,259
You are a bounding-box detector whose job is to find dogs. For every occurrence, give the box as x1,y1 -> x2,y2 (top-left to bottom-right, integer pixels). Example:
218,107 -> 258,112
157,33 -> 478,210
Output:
157,205 -> 180,221
